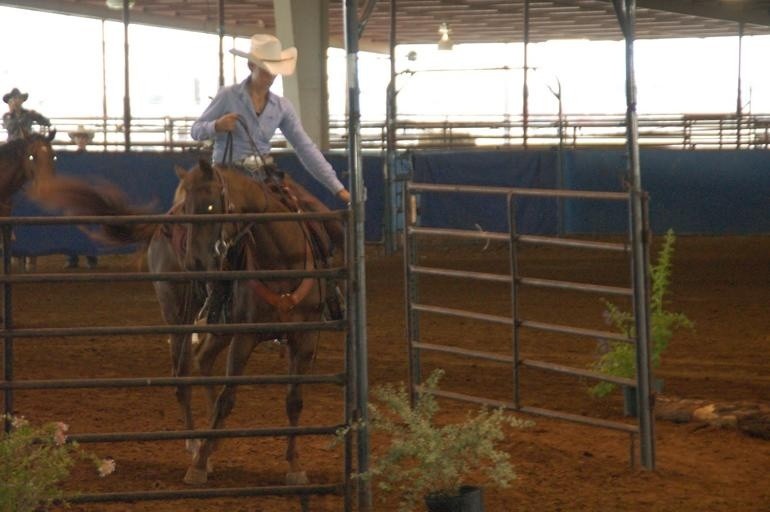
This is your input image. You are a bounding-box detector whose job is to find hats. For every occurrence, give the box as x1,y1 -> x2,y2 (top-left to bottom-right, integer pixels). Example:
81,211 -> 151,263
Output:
229,33 -> 300,75
1,86 -> 30,104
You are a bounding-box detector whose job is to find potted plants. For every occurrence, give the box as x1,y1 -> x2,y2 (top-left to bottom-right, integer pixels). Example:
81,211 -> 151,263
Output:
330,366 -> 537,511
577,228 -> 697,416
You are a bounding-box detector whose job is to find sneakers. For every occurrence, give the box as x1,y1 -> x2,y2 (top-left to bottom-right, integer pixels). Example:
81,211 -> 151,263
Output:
325,286 -> 347,320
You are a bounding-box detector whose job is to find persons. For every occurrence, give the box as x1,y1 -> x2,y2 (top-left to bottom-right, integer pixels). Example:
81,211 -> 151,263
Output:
63,125 -> 104,270
2,89 -> 52,143
188,37 -> 354,322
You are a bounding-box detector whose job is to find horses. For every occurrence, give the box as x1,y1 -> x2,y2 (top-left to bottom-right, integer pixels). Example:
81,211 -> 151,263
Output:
0,126 -> 61,229
26,151 -> 332,490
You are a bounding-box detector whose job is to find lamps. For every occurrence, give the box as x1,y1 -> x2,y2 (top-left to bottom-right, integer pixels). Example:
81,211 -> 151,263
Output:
437,21 -> 454,50
407,51 -> 418,73
